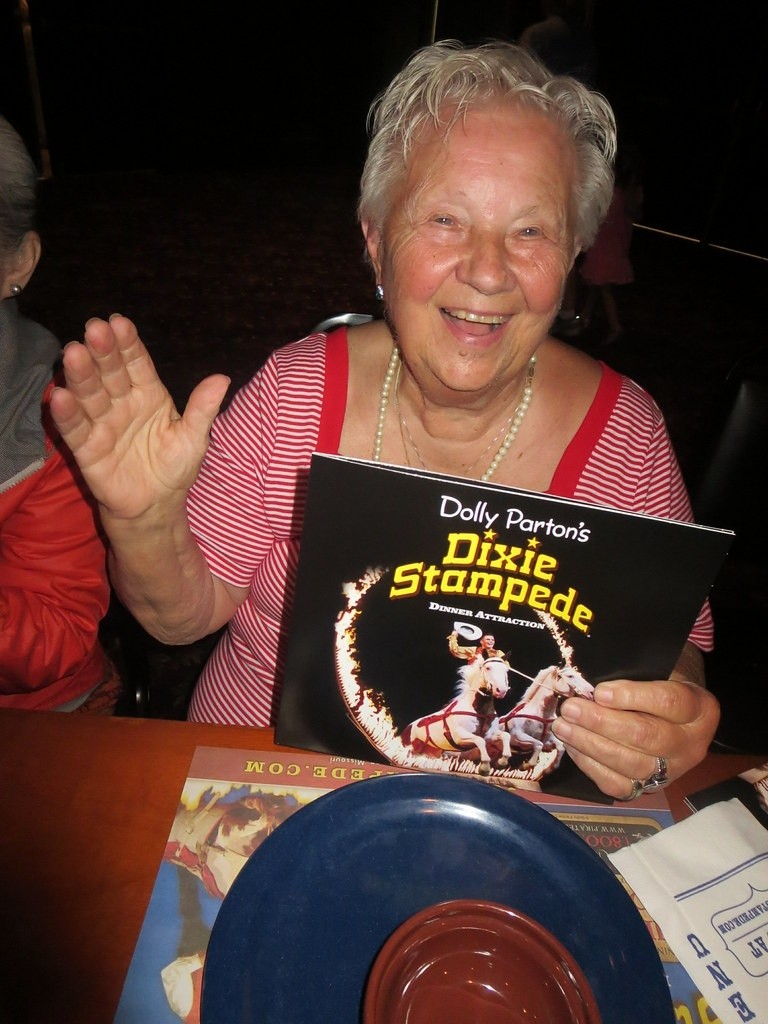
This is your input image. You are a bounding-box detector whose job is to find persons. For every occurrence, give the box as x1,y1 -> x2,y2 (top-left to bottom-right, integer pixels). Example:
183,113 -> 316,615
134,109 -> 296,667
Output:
44,0 -> 719,802
0,105 -> 119,721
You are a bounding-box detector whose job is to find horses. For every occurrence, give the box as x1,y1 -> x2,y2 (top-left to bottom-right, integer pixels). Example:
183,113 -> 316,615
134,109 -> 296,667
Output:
399,648 -> 597,773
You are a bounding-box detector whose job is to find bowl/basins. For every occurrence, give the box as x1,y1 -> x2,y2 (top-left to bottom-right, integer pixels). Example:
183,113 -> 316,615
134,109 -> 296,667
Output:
364,899 -> 602,1024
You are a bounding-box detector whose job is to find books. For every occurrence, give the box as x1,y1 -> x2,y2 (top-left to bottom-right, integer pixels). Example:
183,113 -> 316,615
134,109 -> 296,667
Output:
266,461 -> 733,798
266,452 -> 706,730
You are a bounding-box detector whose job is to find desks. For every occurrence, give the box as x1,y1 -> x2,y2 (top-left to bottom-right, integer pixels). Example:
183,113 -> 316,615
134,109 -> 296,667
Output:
1,711 -> 768,1024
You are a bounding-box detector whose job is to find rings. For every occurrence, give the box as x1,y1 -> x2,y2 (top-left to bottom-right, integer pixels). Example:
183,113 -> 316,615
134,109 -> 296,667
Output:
615,779 -> 640,801
642,757 -> 671,794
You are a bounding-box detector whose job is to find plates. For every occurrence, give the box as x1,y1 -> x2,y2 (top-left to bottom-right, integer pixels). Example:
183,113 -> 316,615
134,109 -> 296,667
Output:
200,773 -> 678,1024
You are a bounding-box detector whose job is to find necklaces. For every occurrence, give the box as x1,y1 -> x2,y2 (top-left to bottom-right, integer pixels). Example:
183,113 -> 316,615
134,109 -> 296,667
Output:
372,346 -> 536,484
